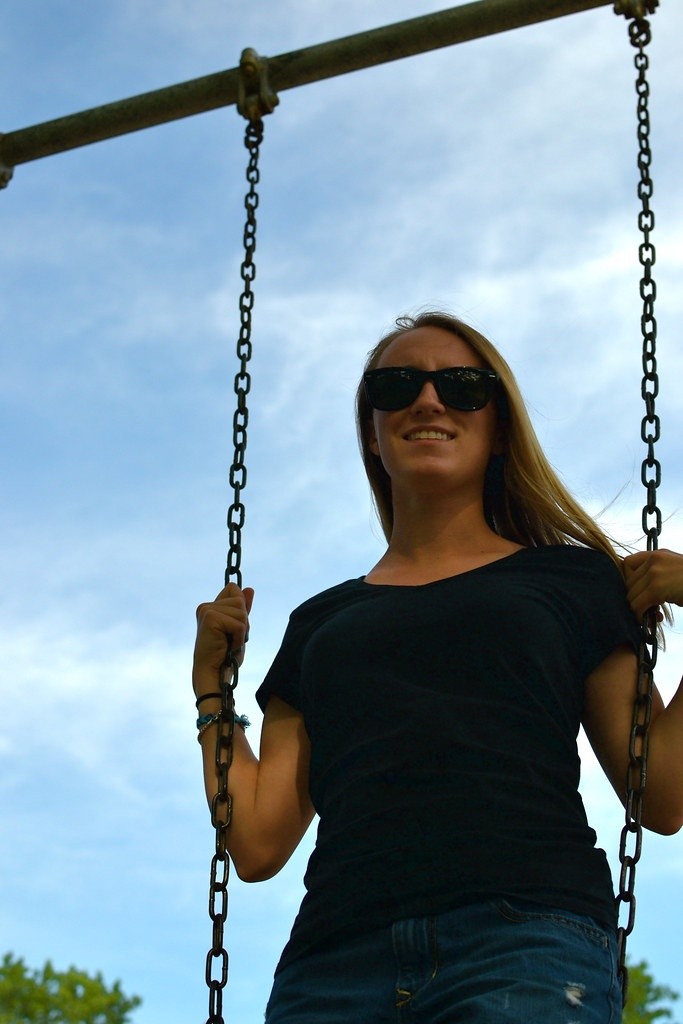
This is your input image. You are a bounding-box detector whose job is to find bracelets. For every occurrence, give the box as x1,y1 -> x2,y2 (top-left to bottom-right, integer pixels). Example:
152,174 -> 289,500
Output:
195,693 -> 235,710
196,710 -> 251,744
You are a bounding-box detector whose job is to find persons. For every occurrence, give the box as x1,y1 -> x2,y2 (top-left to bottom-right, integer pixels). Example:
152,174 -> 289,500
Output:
192,314 -> 683,1024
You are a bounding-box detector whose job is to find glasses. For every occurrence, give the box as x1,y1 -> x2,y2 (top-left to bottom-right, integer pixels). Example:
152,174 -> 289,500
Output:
362,367 -> 498,413
